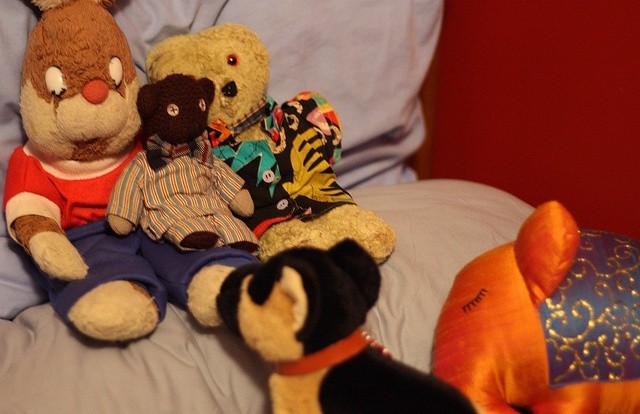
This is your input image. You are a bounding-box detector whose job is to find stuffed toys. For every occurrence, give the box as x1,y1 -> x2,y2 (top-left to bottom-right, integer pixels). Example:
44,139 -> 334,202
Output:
104,75 -> 260,249
216,236 -> 479,414
2,1 -> 261,346
432,202 -> 639,414
143,22 -> 398,264
